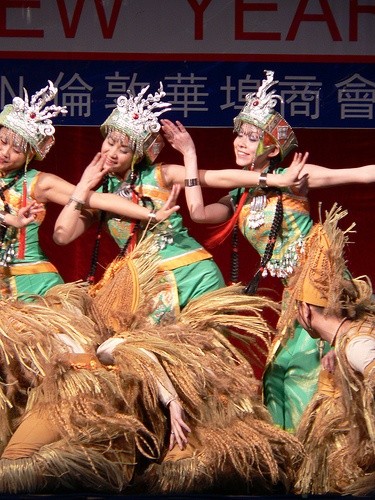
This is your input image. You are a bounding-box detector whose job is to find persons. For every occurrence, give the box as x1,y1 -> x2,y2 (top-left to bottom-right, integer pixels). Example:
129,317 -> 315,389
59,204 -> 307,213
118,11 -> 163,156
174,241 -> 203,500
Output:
260,202 -> 375,500
53,82 -> 227,315
0,80 -> 181,315
86,214 -> 303,500
160,69 -> 375,436
0,278 -> 155,500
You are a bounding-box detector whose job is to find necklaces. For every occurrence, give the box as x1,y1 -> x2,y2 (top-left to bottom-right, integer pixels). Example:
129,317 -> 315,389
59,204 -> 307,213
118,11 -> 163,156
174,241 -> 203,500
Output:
232,166 -> 284,296
89,174 -> 141,284
0,170 -> 33,271
328,316 -> 349,347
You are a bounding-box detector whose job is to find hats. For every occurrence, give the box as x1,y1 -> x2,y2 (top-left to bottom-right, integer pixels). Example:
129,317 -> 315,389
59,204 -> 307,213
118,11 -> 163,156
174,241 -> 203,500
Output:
275,202 -> 373,347
100,81 -> 172,253
204,70 -> 298,248
0,80 -> 68,259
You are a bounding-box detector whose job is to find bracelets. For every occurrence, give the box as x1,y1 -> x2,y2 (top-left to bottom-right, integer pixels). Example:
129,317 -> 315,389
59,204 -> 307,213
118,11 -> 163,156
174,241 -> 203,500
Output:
184,178 -> 199,187
259,172 -> 268,187
66,198 -> 84,211
148,208 -> 158,223
0,211 -> 9,224
